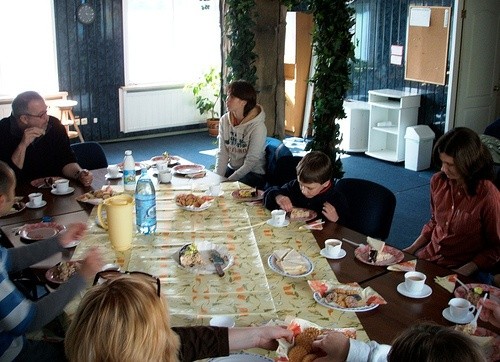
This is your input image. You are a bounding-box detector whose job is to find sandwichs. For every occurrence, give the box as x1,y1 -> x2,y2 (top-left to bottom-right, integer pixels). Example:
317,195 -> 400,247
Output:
274,247 -> 307,275
365,236 -> 386,252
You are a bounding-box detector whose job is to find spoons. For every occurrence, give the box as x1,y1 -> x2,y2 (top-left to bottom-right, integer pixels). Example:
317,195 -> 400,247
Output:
467,293 -> 488,334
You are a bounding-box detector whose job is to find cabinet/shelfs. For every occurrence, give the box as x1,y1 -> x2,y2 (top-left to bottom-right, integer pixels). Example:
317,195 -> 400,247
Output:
334,99 -> 370,152
284,11 -> 313,138
365,89 -> 420,162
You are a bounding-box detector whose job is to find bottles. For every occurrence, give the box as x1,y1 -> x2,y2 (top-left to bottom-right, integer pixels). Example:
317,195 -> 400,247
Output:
134,168 -> 156,239
123,150 -> 137,191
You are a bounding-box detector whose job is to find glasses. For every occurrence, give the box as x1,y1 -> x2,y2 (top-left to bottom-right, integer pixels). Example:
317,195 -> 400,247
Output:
91,269 -> 160,298
18,106 -> 49,119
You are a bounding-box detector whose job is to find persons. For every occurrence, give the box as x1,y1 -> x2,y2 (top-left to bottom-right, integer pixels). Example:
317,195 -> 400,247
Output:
64,274 -> 293,362
213,79 -> 268,190
0,91 -> 94,188
262,152 -> 352,228
401,126 -> 499,285
476,298 -> 500,327
311,322 -> 487,362
0,161 -> 106,362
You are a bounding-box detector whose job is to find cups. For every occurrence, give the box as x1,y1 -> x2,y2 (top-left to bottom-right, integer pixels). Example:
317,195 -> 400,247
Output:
28,193 -> 42,206
97,195 -> 135,252
51,179 -> 69,193
107,165 -> 119,177
404,271 -> 427,293
209,183 -> 222,195
448,298 -> 475,321
158,169 -> 172,184
271,209 -> 286,224
325,239 -> 342,256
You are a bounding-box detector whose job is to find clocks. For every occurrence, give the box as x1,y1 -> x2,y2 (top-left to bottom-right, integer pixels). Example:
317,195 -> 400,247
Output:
77,3 -> 97,24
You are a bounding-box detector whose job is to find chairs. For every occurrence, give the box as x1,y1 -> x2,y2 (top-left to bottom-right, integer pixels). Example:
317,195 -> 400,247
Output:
274,156 -> 303,188
332,177 -> 397,241
70,141 -> 106,170
480,165 -> 500,283
264,137 -> 293,192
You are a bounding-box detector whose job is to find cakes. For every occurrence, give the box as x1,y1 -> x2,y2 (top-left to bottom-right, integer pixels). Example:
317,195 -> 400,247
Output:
179,244 -> 206,267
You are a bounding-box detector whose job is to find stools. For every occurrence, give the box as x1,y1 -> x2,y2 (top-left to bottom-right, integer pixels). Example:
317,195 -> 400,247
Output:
54,99 -> 84,143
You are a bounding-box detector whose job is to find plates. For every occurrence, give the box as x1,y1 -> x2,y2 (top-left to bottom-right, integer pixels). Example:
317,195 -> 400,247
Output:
454,283 -> 500,309
397,281 -> 432,299
267,250 -> 314,277
104,173 -> 123,179
354,245 -> 404,266
207,352 -> 274,362
151,155 -> 180,166
115,162 -> 149,173
231,188 -> 265,202
205,190 -> 225,197
57,227 -> 81,248
275,317 -> 356,362
285,207 -> 317,222
172,164 -> 205,174
18,223 -> 66,240
320,248 -> 346,259
314,285 -> 379,312
173,241 -> 232,275
156,161 -> 167,171
45,260 -> 85,284
26,200 -> 48,208
174,193 -> 215,212
442,307 -> 475,324
210,316 -> 235,328
51,187 -> 74,195
267,219 -> 290,227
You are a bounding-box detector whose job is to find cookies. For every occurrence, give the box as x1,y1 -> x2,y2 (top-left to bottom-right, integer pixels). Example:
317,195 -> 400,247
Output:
176,193 -> 205,207
287,328 -> 355,362
324,289 -> 364,307
80,189 -> 115,200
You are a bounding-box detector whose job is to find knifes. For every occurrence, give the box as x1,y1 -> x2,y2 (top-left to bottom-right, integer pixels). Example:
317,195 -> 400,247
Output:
210,249 -> 225,277
